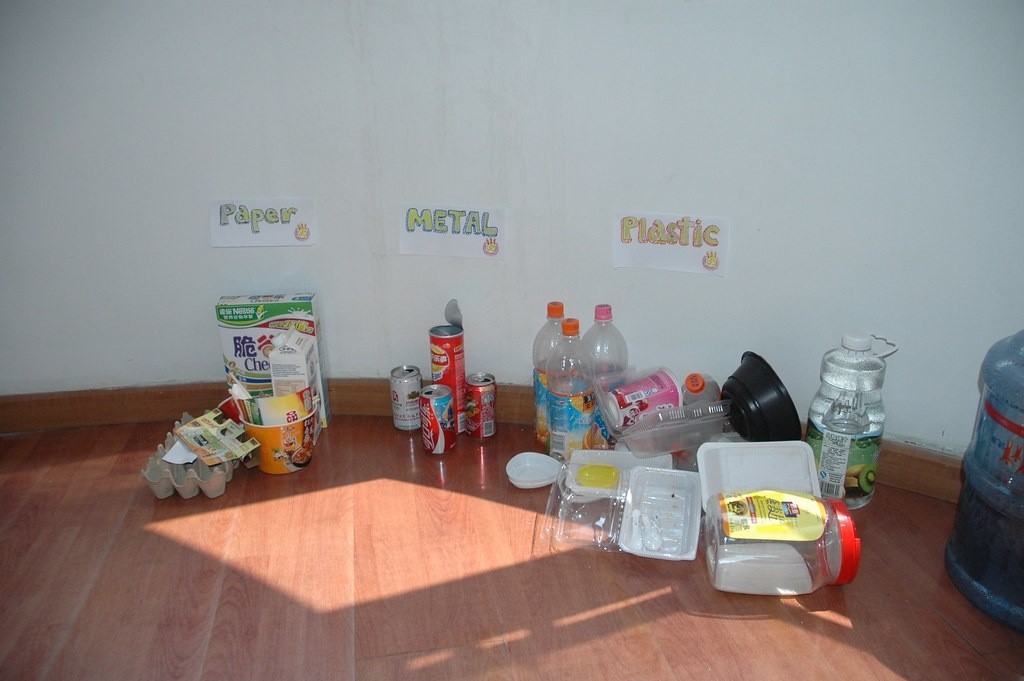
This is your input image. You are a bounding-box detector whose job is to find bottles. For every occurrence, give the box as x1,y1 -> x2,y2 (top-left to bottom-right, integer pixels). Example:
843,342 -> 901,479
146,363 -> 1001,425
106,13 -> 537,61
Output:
706,489 -> 862,595
943,327 -> 1024,634
804,331 -> 899,511
581,304 -> 629,441
545,318 -> 595,469
532,302 -> 564,455
681,373 -> 721,406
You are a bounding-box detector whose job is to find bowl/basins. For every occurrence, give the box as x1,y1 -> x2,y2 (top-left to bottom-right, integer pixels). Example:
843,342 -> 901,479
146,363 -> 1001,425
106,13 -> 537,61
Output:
721,350 -> 803,442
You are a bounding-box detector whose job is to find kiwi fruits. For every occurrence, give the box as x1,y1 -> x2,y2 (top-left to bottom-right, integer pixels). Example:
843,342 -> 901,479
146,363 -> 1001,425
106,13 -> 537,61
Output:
845,462 -> 877,494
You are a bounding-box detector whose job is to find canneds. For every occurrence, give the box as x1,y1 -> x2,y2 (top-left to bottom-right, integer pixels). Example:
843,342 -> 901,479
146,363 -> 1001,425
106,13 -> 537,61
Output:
390,365 -> 422,431
418,384 -> 457,454
464,371 -> 498,439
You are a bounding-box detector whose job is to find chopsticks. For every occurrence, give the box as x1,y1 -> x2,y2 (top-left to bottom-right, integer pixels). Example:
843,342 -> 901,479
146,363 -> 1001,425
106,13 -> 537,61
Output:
310,394 -> 320,405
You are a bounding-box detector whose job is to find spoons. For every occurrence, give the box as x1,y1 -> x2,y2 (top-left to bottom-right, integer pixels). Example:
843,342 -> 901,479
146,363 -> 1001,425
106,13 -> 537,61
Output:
641,513 -> 662,551
628,508 -> 643,551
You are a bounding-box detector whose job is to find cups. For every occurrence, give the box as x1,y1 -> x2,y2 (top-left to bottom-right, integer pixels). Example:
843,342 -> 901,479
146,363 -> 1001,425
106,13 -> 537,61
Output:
217,396 -> 242,425
606,368 -> 682,430
